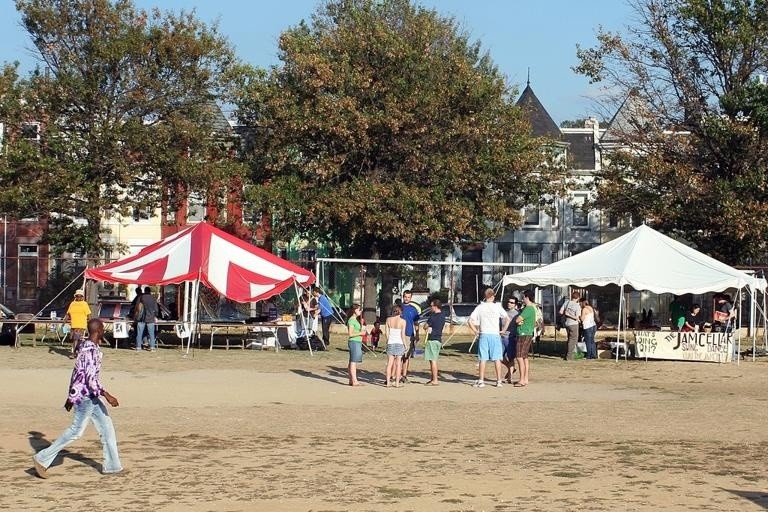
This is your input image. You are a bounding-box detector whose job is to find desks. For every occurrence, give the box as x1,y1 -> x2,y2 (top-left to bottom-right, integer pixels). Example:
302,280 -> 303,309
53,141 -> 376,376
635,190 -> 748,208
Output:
0,318 -> 70,349
633,329 -> 735,363
98,320 -> 177,351
197,321 -> 291,351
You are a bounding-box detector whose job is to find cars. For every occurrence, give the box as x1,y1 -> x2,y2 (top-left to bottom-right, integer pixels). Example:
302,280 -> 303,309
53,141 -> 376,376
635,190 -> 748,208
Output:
414,303 -> 504,325
0,303 -> 18,332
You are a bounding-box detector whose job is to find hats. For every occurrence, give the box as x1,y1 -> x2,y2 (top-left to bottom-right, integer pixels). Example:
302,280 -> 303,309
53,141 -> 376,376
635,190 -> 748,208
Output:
74,290 -> 84,297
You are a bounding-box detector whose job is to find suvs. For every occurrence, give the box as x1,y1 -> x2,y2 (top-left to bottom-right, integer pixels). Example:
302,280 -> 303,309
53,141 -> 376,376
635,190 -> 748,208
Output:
95,299 -> 173,346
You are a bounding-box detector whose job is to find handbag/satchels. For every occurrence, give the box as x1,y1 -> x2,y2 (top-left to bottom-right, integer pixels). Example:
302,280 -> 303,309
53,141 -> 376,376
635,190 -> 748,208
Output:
557,315 -> 567,328
137,302 -> 145,321
594,315 -> 602,328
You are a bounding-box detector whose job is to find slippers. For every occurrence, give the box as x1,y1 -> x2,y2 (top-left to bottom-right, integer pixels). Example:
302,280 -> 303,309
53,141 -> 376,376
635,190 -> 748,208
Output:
502,369 -> 527,386
349,382 -> 363,386
425,380 -> 439,386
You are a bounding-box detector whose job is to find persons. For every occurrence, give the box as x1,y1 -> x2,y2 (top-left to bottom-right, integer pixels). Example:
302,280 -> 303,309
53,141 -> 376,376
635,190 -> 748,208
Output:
559,291 -> 601,361
128,287 -> 159,351
359,319 -> 367,354
423,299 -> 445,386
345,304 -> 366,386
467,288 -> 545,387
300,287 -> 335,350
261,299 -> 277,316
32,318 -> 124,479
384,289 -> 420,386
668,293 -> 737,332
370,322 -> 383,349
61,289 -> 91,359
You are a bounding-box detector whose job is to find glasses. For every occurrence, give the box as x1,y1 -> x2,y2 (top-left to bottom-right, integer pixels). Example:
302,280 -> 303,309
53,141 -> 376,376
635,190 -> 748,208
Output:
508,301 -> 515,304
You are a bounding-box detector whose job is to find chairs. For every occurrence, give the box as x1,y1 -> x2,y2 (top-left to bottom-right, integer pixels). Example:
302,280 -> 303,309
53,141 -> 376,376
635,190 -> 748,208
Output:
14,313 -> 36,348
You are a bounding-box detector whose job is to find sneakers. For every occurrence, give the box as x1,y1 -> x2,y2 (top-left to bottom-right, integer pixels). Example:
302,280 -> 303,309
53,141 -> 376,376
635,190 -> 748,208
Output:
70,351 -> 76,359
102,468 -> 129,474
496,381 -> 503,387
32,456 -> 48,479
384,376 -> 408,387
131,343 -> 155,352
473,380 -> 484,387
